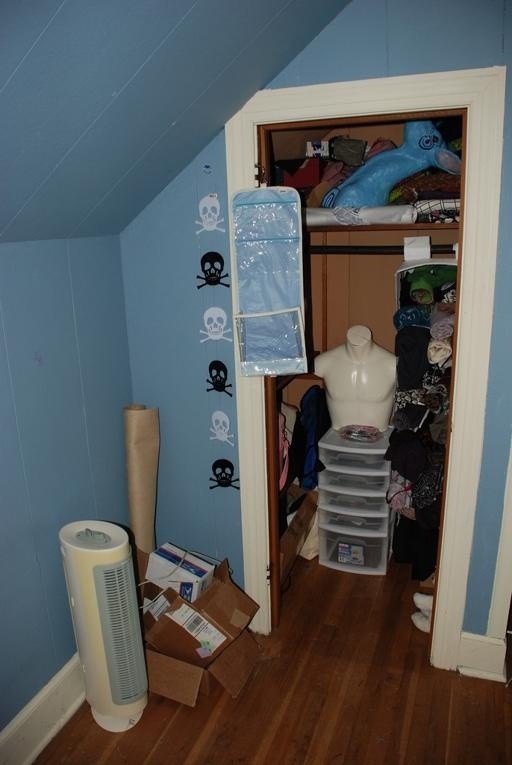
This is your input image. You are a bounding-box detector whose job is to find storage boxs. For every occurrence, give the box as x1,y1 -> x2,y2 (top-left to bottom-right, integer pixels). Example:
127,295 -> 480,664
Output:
140,541 -> 266,707
317,425 -> 395,576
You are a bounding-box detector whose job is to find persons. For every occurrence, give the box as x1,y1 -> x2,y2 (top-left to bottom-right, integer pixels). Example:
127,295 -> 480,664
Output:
313,324 -> 399,433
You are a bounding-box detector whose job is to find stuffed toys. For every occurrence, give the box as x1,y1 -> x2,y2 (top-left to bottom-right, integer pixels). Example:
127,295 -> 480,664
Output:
322,121 -> 462,209
